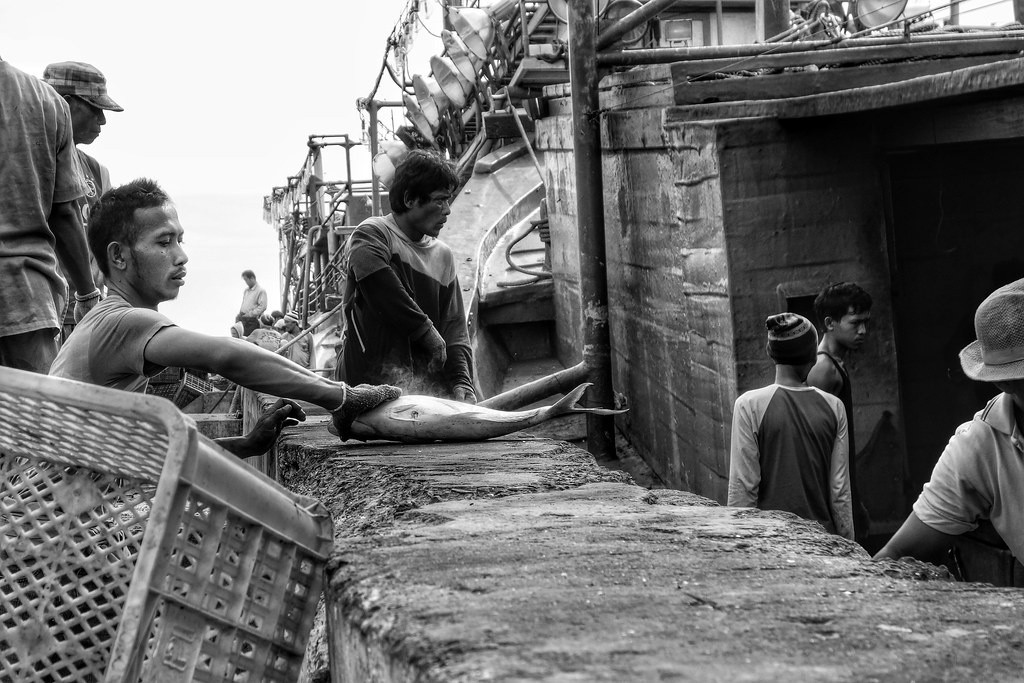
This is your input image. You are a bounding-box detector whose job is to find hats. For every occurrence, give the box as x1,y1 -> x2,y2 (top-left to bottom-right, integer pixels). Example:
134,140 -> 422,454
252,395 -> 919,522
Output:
44,61 -> 123,111
959,277 -> 1023,382
765,312 -> 819,365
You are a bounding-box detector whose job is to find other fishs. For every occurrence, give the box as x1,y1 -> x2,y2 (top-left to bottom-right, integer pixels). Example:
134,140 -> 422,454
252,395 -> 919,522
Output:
327,382 -> 630,444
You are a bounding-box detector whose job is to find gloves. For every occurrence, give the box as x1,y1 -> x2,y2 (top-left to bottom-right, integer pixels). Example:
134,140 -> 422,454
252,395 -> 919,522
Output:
419,324 -> 448,374
330,382 -> 402,443
453,383 -> 476,406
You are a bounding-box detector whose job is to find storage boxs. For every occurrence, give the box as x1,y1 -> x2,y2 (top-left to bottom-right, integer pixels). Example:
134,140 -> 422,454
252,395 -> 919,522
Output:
0,364 -> 334,683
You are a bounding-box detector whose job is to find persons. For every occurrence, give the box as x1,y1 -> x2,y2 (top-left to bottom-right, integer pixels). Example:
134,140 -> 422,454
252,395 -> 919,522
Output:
45,178 -> 402,458
725,312 -> 855,539
332,149 -> 477,405
805,280 -> 874,540
869,277 -> 1024,563
229,311 -> 342,381
40,59 -> 124,346
235,269 -> 268,336
0,55 -> 102,373
